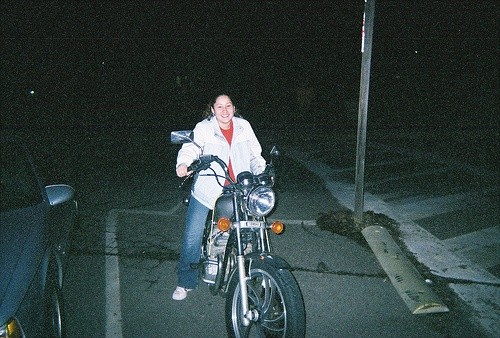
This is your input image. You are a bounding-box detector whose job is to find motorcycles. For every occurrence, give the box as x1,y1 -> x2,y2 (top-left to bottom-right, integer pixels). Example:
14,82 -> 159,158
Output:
169,129 -> 307,338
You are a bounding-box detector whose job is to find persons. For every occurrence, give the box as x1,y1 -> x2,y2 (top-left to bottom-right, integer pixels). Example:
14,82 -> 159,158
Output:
172,93 -> 267,301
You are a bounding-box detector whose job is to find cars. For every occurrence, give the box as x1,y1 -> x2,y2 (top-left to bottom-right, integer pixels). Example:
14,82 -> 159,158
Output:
0,129 -> 80,338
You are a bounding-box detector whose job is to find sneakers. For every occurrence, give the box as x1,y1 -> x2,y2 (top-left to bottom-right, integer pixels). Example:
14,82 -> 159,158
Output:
171,285 -> 196,302
